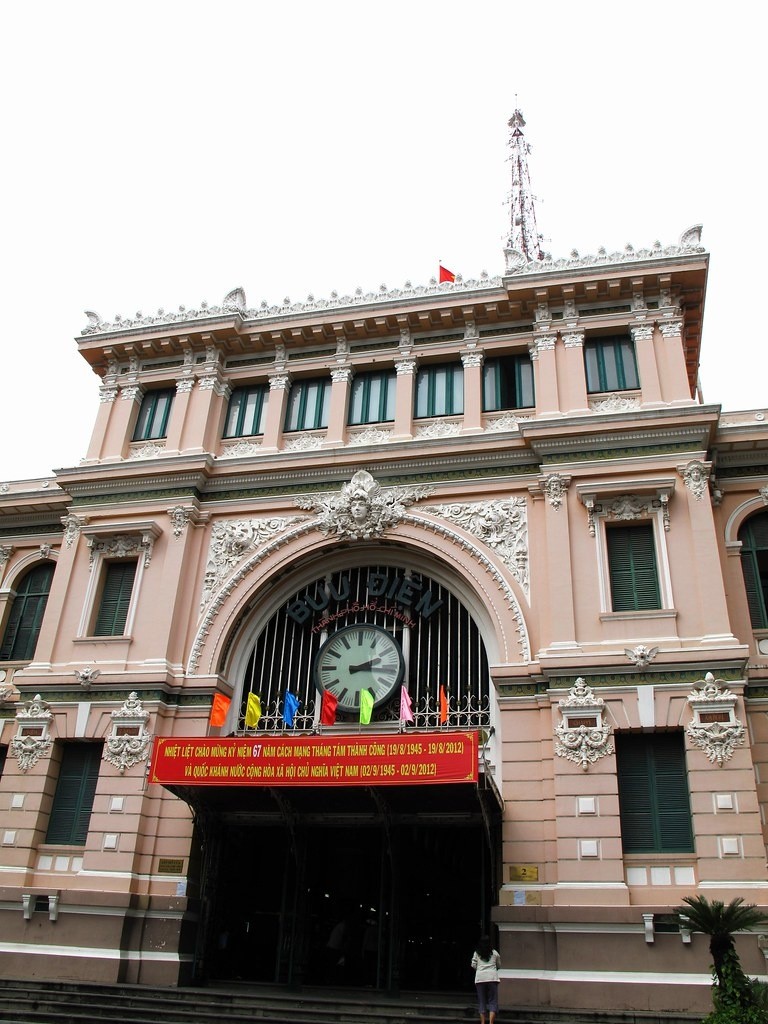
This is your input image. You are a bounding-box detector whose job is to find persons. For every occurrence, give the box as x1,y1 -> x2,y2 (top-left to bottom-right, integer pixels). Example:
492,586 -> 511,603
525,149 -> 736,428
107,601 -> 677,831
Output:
470,934 -> 501,1024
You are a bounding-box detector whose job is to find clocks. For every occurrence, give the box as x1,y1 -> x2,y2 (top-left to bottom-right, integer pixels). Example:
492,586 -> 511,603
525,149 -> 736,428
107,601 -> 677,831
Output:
314,623 -> 404,712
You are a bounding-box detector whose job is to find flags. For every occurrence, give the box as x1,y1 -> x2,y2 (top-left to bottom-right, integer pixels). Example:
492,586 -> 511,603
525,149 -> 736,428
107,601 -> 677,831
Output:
207,684 -> 449,728
440,265 -> 455,284
